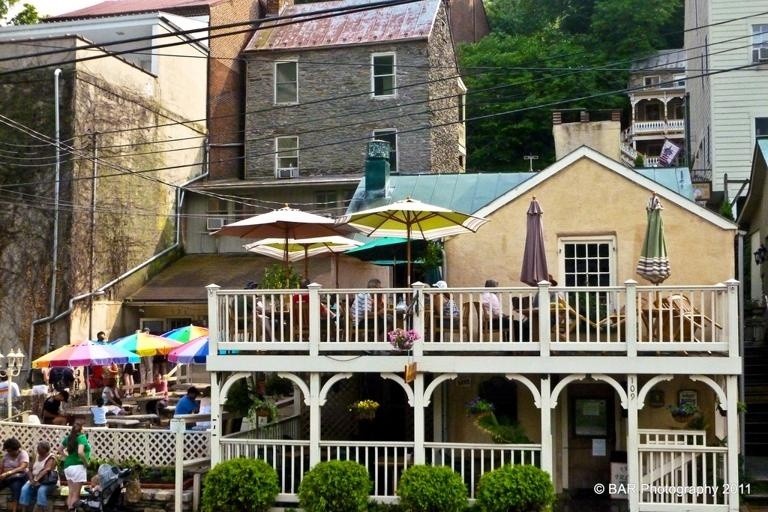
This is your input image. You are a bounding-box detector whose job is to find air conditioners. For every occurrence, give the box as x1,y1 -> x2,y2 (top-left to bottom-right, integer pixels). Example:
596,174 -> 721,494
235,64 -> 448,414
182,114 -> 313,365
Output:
757,47 -> 767,61
207,217 -> 224,229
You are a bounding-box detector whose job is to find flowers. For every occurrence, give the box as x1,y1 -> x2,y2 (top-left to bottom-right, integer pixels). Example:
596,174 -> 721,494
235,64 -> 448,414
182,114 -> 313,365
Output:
388,328 -> 421,346
666,400 -> 697,414
347,399 -> 380,409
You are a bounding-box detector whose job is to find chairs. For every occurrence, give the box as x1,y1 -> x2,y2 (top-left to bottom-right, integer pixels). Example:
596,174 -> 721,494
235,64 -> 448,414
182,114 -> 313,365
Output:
230,281 -> 724,356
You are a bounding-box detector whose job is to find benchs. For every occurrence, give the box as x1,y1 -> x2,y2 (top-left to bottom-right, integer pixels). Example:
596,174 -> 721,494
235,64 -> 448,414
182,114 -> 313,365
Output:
54,379 -> 212,430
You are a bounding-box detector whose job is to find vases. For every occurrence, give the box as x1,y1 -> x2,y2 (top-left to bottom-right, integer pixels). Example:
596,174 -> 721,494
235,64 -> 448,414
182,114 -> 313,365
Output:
397,340 -> 413,350
356,408 -> 376,420
670,412 -> 689,423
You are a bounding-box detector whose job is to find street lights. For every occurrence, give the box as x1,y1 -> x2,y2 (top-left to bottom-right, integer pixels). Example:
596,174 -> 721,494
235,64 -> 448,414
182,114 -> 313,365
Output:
676,93 -> 690,164
1,348 -> 25,417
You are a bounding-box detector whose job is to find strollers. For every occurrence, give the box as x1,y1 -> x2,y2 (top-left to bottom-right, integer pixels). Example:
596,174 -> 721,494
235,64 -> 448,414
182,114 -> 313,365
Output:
73,463 -> 131,512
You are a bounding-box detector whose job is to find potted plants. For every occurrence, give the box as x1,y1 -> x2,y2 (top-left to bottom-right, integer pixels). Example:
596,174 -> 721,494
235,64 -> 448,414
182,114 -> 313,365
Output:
246,396 -> 279,427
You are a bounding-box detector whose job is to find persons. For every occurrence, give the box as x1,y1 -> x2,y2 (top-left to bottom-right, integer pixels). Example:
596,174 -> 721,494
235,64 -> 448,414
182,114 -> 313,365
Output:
0,326 -> 267,512
231,273 -> 576,342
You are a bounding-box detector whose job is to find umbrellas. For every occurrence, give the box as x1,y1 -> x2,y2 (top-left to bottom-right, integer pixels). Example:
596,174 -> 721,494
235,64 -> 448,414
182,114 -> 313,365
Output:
637,191 -> 671,342
520,196 -> 549,287
208,195 -> 490,315
